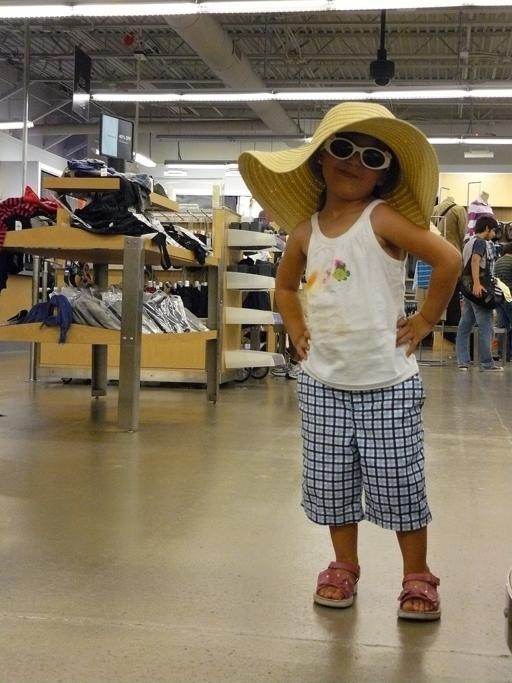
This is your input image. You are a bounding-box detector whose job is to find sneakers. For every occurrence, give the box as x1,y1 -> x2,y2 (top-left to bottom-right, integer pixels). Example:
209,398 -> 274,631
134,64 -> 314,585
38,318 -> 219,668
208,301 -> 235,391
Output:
284,362 -> 300,379
458,361 -> 505,372
270,360 -> 295,376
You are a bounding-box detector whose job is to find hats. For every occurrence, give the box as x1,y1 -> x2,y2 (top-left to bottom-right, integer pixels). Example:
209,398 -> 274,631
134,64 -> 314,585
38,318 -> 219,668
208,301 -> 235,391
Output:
234,99 -> 443,240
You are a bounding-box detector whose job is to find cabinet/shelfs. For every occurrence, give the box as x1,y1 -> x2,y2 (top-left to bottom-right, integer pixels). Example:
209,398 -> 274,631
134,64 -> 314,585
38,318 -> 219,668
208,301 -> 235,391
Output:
1,176 -> 286,433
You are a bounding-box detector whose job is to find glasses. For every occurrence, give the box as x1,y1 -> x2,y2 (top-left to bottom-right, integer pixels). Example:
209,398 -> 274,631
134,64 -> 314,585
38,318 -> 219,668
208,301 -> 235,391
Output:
324,135 -> 394,171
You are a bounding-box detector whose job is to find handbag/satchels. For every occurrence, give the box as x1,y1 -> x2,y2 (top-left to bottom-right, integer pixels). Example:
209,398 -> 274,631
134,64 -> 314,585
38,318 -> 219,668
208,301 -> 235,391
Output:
456,268 -> 504,311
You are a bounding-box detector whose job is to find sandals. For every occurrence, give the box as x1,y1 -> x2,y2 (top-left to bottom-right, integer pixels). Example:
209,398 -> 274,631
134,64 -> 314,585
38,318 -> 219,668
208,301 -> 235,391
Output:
395,570 -> 443,622
312,558 -> 361,609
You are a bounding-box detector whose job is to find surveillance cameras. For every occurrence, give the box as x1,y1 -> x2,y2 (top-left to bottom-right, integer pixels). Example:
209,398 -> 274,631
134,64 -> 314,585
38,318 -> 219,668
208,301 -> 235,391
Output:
368,60 -> 395,85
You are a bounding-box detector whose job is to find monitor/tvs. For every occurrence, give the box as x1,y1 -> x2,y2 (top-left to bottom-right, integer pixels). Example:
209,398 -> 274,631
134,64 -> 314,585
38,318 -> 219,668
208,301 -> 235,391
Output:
99,111 -> 135,162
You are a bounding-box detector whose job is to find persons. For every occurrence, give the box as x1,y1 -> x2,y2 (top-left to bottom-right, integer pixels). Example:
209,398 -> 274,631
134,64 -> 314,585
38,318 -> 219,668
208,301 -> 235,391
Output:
273,102 -> 467,622
454,215 -> 503,372
491,243 -> 511,361
